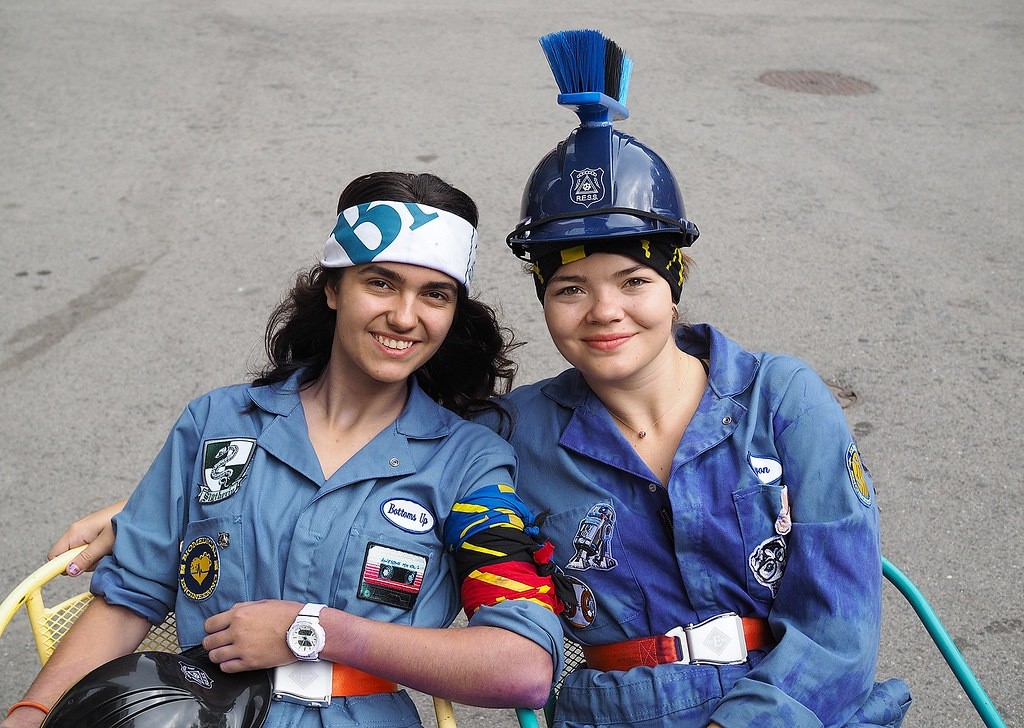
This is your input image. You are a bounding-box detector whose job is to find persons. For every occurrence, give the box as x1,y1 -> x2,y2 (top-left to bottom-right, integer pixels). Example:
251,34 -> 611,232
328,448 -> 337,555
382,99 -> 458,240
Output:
2,170 -> 558,728
45,120 -> 901,728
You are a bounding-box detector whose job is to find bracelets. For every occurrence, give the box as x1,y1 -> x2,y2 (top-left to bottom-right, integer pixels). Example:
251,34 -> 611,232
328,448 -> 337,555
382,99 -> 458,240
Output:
4,701 -> 48,719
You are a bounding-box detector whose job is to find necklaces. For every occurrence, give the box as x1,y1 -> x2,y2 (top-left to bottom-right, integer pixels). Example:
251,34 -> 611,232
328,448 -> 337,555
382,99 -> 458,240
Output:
598,351 -> 692,440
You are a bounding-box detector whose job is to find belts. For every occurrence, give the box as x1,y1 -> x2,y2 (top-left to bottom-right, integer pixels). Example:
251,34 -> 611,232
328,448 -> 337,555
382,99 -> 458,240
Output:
274,659 -> 398,708
582,612 -> 774,673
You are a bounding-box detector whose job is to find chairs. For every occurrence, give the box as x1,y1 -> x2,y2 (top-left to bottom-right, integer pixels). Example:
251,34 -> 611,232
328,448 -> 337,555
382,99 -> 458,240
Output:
0,544 -> 458,728
515,554 -> 1010,728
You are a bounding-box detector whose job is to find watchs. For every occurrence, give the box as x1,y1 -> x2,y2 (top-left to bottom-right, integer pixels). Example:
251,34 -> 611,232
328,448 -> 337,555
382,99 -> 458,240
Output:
284,602 -> 330,662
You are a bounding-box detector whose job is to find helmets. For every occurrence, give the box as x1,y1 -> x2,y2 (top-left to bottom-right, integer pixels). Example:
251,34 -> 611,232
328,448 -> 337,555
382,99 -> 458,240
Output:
505,126 -> 698,257
39,644 -> 274,728
